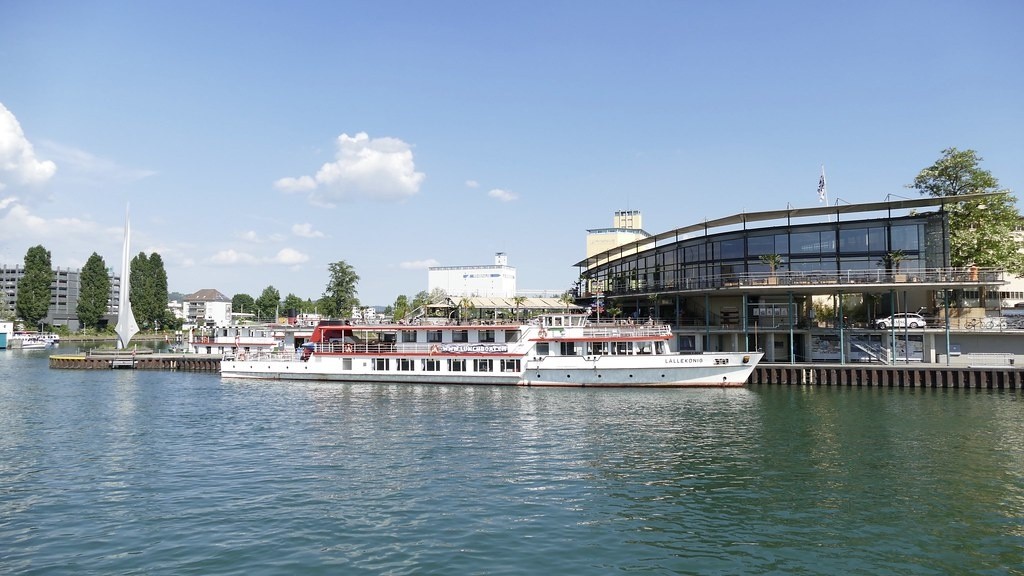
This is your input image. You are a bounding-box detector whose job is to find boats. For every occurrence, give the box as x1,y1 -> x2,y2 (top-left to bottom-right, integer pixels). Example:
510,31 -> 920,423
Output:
8,330 -> 61,350
301,342 -> 315,350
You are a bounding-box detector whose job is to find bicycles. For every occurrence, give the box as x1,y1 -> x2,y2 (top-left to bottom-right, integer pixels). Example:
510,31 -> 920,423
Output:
964,317 -> 1008,330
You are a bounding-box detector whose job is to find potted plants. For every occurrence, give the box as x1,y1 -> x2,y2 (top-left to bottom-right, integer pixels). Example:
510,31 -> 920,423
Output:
759,254 -> 781,285
882,249 -> 909,282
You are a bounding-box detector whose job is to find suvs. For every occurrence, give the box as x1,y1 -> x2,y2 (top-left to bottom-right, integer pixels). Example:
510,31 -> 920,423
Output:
870,306 -> 927,330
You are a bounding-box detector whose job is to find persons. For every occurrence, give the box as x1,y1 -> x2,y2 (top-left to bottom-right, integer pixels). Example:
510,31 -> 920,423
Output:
648,315 -> 652,322
628,317 -> 634,325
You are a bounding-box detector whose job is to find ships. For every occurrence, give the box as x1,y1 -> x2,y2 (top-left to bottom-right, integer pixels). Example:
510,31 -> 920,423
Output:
218,312 -> 767,389
